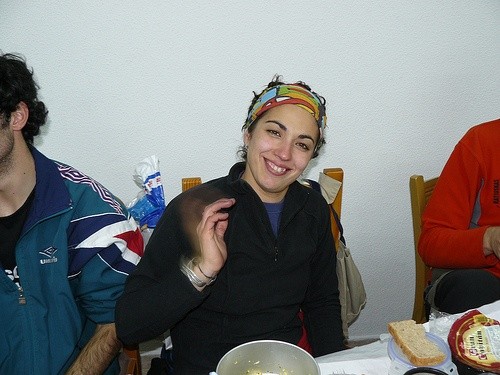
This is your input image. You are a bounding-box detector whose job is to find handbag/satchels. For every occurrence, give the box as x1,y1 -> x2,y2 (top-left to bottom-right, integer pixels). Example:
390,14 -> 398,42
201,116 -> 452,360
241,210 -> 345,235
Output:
329,203 -> 368,327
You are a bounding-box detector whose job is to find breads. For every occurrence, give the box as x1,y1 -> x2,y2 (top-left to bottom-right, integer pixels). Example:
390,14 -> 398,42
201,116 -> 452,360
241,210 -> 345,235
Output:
387,319 -> 445,366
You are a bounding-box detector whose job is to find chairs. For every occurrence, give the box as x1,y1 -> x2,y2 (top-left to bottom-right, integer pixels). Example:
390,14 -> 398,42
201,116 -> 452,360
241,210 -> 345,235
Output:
410,173 -> 439,325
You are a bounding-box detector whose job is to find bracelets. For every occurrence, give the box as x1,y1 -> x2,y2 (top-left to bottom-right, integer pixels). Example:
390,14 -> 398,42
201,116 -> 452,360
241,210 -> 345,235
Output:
198,259 -> 212,278
187,256 -> 217,287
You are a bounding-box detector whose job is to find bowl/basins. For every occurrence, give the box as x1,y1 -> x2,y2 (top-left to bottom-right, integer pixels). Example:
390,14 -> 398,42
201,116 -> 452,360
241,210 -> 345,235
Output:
216,340 -> 320,375
387,332 -> 458,375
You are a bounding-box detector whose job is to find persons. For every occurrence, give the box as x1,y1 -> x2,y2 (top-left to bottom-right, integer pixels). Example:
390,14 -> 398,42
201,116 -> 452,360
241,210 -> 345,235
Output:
0,47 -> 146,375
418,119 -> 500,314
115,73 -> 350,375
